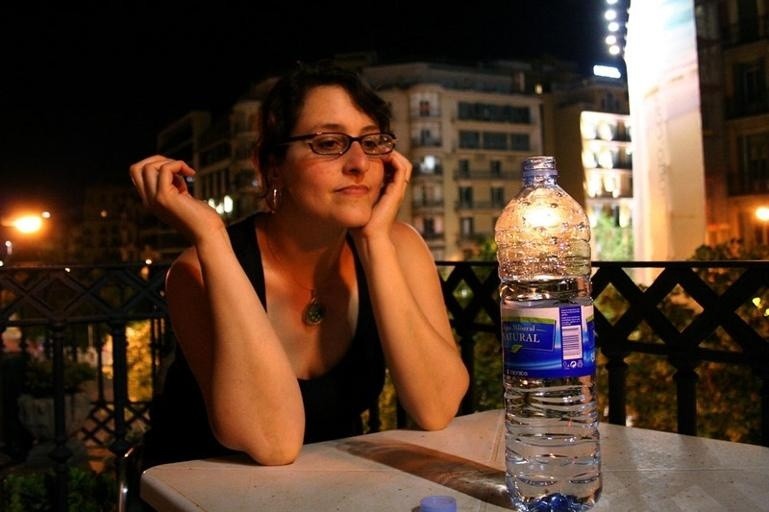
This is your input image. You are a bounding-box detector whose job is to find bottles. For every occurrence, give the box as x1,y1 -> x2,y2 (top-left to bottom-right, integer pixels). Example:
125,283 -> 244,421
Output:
494,156 -> 605,512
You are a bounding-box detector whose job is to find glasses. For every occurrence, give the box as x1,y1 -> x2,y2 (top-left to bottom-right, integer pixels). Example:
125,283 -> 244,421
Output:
278,131 -> 398,156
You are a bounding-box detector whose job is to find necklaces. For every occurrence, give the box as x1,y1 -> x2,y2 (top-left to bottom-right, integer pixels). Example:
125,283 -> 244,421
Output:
265,212 -> 347,326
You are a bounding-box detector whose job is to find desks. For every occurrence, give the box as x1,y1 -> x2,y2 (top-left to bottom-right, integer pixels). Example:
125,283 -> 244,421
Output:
137,410 -> 768,507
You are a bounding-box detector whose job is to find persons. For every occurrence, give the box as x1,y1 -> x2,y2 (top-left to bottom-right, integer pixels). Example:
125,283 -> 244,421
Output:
125,62 -> 470,512
